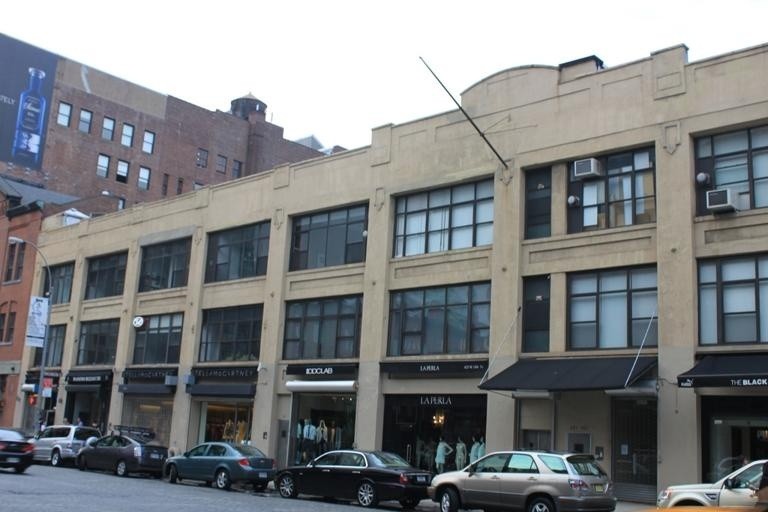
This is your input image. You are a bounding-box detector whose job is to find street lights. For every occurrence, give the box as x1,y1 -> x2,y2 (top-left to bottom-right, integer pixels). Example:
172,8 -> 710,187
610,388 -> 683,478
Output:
8,236 -> 53,435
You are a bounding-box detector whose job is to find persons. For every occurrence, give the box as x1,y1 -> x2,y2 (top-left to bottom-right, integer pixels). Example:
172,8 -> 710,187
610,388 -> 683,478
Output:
62,415 -> 98,426
222,418 -> 251,444
750,461 -> 767,511
295,419 -> 342,464
414,434 -> 485,474
733,455 -> 748,468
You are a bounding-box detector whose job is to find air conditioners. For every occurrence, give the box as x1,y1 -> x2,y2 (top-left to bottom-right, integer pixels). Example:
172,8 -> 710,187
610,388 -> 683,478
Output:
706,188 -> 739,212
573,157 -> 603,179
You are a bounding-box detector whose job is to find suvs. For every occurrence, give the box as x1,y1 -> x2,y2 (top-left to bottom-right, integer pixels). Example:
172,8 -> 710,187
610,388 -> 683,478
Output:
658,460 -> 766,508
426,450 -> 616,510
27,425 -> 104,465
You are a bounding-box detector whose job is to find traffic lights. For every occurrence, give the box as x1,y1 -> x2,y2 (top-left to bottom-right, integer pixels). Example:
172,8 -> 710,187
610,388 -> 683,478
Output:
29,396 -> 37,404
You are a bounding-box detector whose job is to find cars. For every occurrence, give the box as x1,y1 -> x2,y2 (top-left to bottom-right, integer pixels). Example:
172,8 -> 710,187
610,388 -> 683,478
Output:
74,434 -> 169,477
275,450 -> 433,509
0,427 -> 34,474
162,442 -> 276,491
